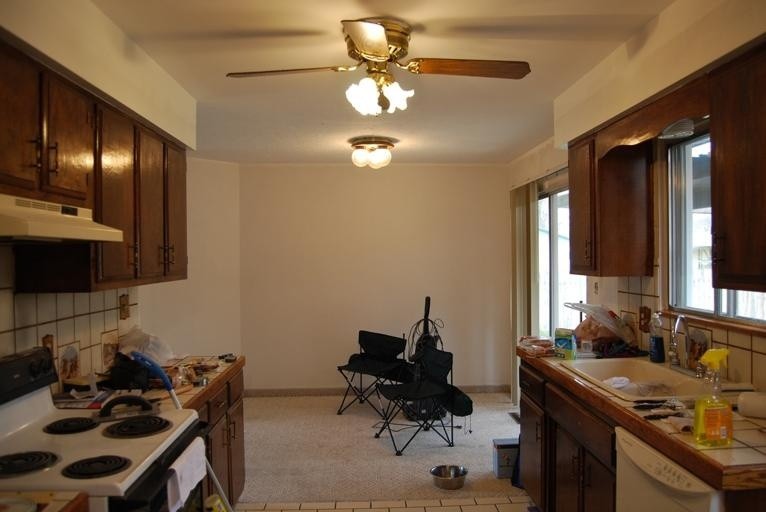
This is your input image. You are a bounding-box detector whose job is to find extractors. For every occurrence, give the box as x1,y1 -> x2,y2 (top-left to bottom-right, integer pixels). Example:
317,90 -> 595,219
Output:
0,194 -> 124,244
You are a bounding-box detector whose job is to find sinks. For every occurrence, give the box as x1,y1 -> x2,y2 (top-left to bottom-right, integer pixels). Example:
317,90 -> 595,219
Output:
558,355 -> 720,404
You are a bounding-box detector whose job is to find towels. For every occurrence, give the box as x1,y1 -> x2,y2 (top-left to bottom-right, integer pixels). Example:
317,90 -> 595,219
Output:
163,435 -> 210,512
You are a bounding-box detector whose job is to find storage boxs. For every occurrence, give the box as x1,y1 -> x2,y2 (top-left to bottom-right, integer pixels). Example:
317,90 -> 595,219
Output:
491,438 -> 519,480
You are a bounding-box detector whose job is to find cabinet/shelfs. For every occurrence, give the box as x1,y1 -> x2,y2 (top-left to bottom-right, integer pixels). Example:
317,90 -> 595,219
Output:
199,367 -> 245,510
0,27 -> 96,210
96,103 -> 136,291
518,358 -> 553,512
543,382 -> 614,511
567,99 -> 654,276
136,121 -> 187,287
709,31 -> 765,292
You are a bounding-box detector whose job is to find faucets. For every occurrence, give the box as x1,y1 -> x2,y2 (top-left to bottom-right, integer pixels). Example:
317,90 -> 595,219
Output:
673,314 -> 692,369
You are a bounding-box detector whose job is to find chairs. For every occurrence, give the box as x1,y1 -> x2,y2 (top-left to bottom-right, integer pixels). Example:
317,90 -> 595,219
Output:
374,345 -> 453,456
336,330 -> 410,420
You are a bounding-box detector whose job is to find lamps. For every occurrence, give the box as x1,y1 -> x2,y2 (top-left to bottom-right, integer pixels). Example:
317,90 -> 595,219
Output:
350,136 -> 392,170
339,17 -> 415,116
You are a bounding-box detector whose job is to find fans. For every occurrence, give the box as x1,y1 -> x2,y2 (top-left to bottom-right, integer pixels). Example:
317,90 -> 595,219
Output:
225,18 -> 531,79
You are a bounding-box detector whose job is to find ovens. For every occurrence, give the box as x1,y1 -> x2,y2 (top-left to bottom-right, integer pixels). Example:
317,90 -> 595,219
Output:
89,418 -> 213,511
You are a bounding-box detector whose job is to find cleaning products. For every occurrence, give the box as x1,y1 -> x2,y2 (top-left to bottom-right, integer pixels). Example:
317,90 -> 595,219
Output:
692,346 -> 735,450
648,312 -> 666,363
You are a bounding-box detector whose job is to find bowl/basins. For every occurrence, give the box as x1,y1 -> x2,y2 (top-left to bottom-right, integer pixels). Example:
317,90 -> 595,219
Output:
429,463 -> 470,489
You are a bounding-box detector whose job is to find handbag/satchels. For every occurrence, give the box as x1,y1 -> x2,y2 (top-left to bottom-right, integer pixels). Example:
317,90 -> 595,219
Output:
109,352 -> 149,389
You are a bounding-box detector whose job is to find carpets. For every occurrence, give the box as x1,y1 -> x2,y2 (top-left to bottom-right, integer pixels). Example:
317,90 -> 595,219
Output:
237,390 -> 531,504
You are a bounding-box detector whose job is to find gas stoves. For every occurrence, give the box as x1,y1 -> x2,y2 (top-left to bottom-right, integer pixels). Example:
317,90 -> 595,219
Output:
0,409 -> 198,496
